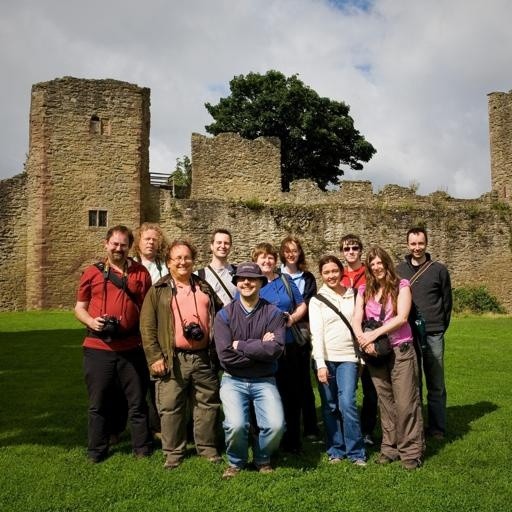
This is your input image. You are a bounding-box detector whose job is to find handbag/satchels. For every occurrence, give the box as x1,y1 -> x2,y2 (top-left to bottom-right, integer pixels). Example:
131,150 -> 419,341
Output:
289,317 -> 313,348
413,317 -> 428,351
357,317 -> 395,368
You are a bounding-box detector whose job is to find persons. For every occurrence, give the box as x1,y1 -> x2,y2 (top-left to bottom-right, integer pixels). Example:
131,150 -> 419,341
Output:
395,228 -> 451,440
109,222 -> 169,445
352,247 -> 426,469
75,226 -> 151,463
277,236 -> 318,439
214,263 -> 286,477
140,239 -> 223,468
340,234 -> 377,445
309,256 -> 367,465
193,229 -> 237,440
234,242 -> 308,453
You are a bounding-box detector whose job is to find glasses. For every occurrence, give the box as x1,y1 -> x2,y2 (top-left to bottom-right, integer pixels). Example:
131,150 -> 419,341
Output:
342,246 -> 362,251
169,257 -> 193,262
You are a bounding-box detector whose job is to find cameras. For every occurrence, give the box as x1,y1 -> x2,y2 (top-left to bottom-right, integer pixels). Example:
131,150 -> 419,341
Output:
99,316 -> 119,338
183,322 -> 205,341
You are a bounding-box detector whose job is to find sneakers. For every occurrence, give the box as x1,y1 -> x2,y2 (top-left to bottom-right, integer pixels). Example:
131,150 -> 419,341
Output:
253,461 -> 273,473
108,434 -> 119,446
199,454 -> 224,464
151,429 -> 162,441
132,446 -> 153,460
401,459 -> 419,470
373,453 -> 401,464
362,434 -> 375,445
163,455 -> 188,469
353,459 -> 368,466
329,458 -> 341,464
423,428 -> 446,441
86,447 -> 108,466
304,434 -> 327,446
221,466 -> 241,481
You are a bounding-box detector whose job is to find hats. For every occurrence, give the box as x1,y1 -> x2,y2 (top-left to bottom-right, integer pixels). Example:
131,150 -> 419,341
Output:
231,262 -> 268,289
